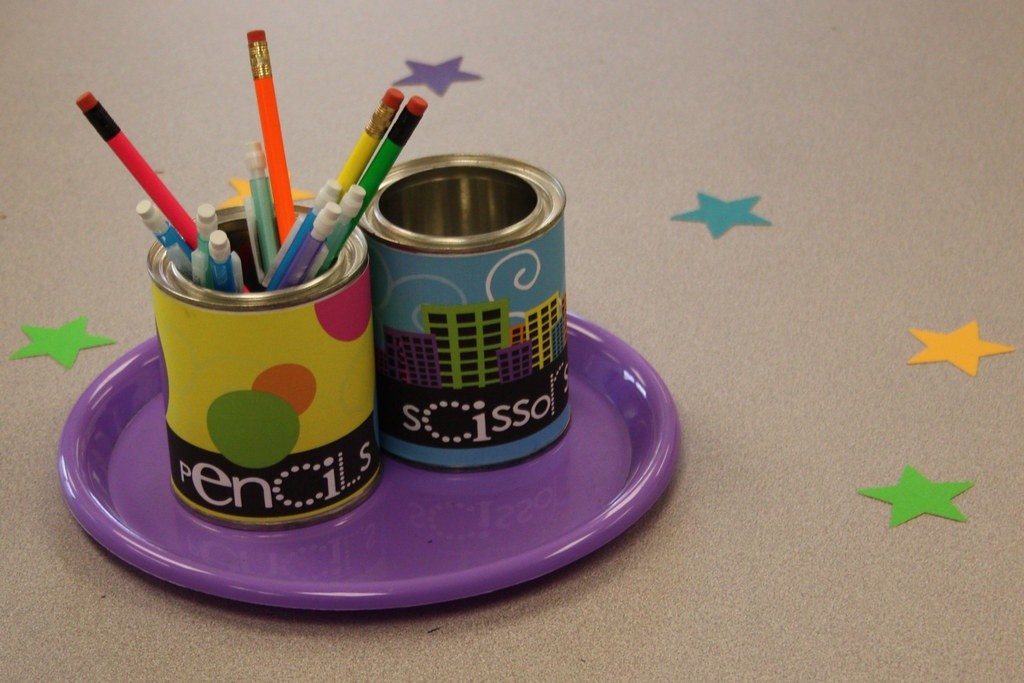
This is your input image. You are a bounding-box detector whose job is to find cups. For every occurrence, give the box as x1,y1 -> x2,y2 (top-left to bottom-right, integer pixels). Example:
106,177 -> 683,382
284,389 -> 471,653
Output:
356,155 -> 571,468
147,204 -> 381,531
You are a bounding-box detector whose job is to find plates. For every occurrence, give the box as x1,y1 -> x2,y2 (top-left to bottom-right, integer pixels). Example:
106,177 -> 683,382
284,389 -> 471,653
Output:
59,312 -> 679,609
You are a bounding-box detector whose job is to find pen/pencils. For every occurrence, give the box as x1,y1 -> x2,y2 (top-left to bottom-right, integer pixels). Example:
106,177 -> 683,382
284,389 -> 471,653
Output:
136,200 -> 192,274
191,204 -> 218,290
209,230 -> 243,293
76,91 -> 250,292
243,142 -> 279,284
247,30 -> 298,247
276,201 -> 342,289
337,88 -> 405,192
336,96 -> 428,259
315,185 -> 366,277
262,180 -> 343,292
